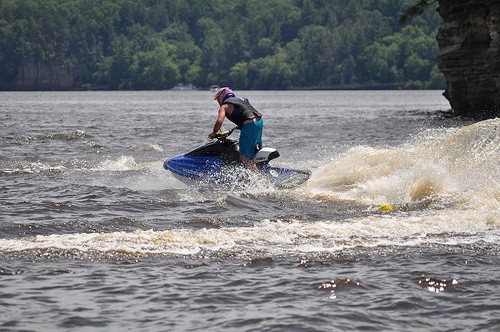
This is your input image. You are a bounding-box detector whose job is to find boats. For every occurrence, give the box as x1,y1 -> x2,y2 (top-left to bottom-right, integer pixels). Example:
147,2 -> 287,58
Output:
163,126 -> 311,196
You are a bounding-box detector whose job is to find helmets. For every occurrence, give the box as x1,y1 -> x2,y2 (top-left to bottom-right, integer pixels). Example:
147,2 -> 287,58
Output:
214,87 -> 233,105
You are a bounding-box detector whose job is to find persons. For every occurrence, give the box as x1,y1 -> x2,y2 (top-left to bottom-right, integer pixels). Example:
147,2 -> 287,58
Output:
209,87 -> 263,173
